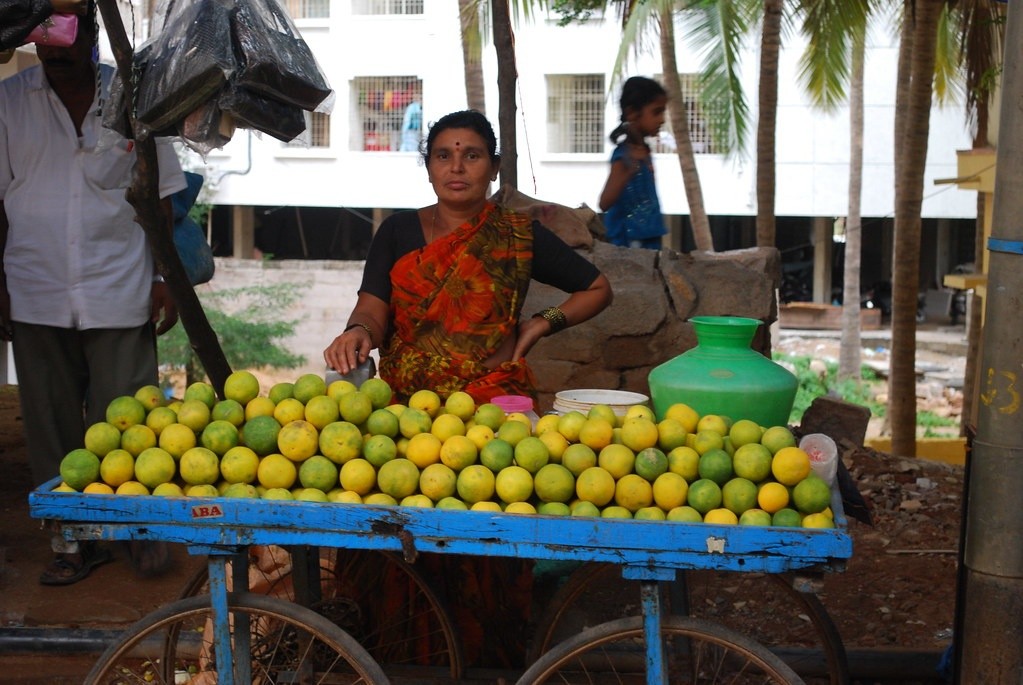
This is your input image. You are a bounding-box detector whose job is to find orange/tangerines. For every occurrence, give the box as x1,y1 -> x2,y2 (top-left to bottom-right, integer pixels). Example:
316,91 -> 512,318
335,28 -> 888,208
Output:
49,368 -> 834,526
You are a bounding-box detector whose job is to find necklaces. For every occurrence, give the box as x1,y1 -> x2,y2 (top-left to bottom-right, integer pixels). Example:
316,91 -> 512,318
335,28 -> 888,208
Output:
429,205 -> 436,243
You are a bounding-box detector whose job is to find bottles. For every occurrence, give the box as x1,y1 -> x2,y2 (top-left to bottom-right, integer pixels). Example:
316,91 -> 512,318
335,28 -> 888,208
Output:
491,395 -> 538,433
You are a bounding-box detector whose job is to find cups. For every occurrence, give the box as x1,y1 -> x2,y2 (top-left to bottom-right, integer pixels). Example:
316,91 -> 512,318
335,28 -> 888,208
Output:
800,433 -> 837,485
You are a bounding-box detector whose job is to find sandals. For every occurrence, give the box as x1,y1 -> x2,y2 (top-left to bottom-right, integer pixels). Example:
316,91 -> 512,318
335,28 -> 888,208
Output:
40,540 -> 111,584
126,539 -> 171,574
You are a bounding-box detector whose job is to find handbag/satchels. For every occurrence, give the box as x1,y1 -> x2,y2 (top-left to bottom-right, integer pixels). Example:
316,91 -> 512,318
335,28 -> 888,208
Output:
101,0 -> 332,145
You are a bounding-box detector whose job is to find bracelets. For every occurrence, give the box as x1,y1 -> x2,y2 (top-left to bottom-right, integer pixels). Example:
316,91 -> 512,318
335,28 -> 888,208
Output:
531,306 -> 568,337
343,322 -> 374,338
153,274 -> 165,283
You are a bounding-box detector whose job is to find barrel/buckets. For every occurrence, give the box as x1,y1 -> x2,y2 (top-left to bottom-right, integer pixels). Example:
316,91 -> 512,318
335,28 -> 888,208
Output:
553,388 -> 650,421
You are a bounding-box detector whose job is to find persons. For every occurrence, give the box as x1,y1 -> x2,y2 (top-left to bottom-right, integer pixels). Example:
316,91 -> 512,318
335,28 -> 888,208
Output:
605,75 -> 671,249
0,0 -> 182,588
322,109 -> 614,685
